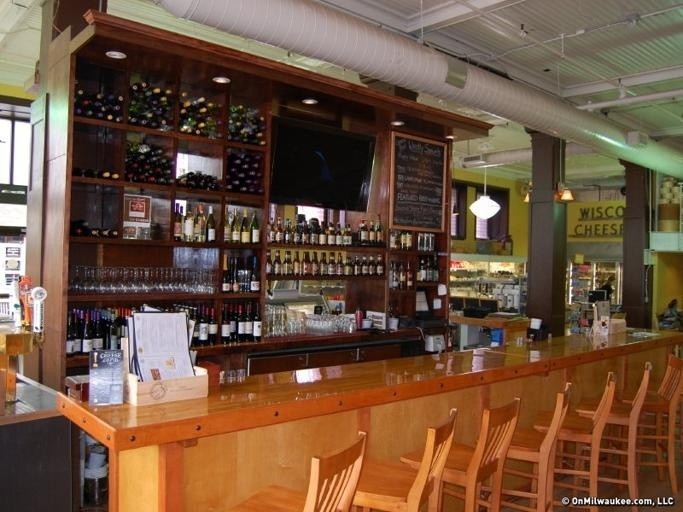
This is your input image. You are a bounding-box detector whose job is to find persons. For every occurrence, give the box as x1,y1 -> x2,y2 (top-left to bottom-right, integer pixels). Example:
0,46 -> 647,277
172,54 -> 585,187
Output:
664,298 -> 681,328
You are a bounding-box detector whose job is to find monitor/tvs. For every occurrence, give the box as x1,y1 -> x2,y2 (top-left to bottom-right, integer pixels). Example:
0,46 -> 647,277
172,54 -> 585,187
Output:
268,115 -> 377,212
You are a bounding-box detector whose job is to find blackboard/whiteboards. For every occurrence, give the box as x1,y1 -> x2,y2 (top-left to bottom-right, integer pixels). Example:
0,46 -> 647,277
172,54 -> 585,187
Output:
388,131 -> 448,233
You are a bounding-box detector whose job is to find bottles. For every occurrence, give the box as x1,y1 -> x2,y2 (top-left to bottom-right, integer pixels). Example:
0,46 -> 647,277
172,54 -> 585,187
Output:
369,219 -> 377,246
265,249 -> 274,275
273,250 -> 282,275
359,218 -> 369,245
283,251 -> 293,276
228,104 -> 269,146
293,250 -> 300,276
174,203 -> 182,242
128,80 -> 175,131
240,208 -> 251,243
150,303 -> 218,346
345,255 -> 353,276
327,253 -> 336,275
67,304 -> 137,357
231,208 -> 242,242
354,307 -> 362,330
368,255 -> 376,276
185,204 -> 206,242
376,253 -> 384,276
225,148 -> 265,196
222,254 -> 261,293
354,256 -> 361,275
250,210 -> 260,242
125,142 -> 177,184
302,252 -> 311,276
179,206 -> 184,242
361,254 -> 370,276
224,207 -> 232,243
265,214 -> 352,246
319,252 -> 328,276
71,219 -> 119,237
179,91 -> 223,140
390,229 -> 436,251
336,252 -> 345,275
205,206 -> 216,242
73,87 -> 124,123
311,252 -> 320,276
221,301 -> 262,344
74,164 -> 121,180
389,257 -> 414,290
177,171 -> 223,191
416,253 -> 440,282
376,212 -> 386,246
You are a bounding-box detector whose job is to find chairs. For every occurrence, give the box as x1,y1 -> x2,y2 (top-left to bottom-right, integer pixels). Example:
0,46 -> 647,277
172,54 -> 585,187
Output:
492,379 -> 574,509
560,360 -> 654,512
348,406 -> 459,512
605,352 -> 683,512
238,427 -> 372,509
522,369 -> 617,506
401,395 -> 521,512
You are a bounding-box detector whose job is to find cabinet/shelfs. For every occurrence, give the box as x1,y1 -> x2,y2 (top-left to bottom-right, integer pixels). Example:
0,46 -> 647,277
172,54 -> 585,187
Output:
60,9 -> 495,382
450,253 -> 528,320
564,259 -> 622,315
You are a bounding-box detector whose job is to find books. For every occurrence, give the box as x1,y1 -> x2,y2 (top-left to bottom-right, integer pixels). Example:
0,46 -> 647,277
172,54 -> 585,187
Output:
126,304 -> 199,381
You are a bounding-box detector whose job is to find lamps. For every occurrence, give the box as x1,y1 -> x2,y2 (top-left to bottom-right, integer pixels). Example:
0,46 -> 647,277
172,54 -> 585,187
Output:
302,97 -> 316,104
213,74 -> 232,84
387,119 -> 405,128
559,188 -> 574,203
445,126 -> 457,142
468,134 -> 502,221
106,45 -> 129,60
